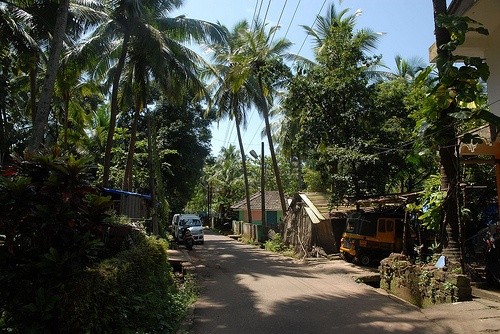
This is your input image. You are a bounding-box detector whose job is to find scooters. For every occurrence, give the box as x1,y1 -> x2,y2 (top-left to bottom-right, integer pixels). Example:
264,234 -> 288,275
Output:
180,225 -> 194,252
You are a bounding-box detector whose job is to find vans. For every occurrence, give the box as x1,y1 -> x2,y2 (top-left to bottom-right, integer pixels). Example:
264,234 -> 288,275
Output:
169,214 -> 179,238
174,214 -> 206,245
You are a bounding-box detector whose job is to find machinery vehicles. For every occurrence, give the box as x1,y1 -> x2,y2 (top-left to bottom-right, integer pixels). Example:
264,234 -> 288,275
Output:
340,208 -> 404,267
